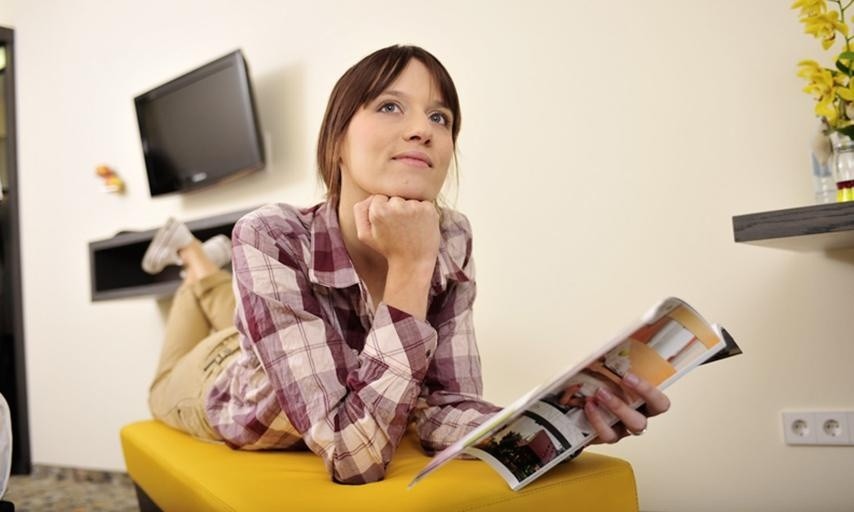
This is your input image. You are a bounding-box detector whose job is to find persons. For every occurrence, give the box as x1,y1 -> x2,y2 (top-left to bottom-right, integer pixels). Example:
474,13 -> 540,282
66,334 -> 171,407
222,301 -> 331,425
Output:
140,45 -> 672,485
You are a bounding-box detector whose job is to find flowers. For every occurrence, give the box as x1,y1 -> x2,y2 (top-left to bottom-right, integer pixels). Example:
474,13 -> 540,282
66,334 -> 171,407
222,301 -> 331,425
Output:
790,1 -> 854,144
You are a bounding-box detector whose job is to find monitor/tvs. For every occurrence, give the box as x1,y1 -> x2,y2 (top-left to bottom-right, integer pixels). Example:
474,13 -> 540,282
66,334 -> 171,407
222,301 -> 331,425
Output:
133,47 -> 266,199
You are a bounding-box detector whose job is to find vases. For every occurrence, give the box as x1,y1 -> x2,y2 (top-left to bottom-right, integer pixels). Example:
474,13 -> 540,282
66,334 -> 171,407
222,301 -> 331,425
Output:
834,146 -> 854,203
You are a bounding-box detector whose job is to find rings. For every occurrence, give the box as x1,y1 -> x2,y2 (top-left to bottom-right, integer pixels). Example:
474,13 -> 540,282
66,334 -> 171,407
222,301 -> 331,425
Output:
623,423 -> 649,436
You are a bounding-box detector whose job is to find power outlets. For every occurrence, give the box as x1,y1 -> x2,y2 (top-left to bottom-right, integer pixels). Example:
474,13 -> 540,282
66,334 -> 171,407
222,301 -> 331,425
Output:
780,410 -> 854,446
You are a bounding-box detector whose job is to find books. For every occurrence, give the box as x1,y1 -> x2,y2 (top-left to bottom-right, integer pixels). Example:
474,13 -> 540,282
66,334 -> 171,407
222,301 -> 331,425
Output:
410,295 -> 742,492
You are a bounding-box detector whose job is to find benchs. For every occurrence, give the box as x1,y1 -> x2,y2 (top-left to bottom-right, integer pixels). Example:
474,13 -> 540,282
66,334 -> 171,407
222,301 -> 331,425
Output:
120,419 -> 642,511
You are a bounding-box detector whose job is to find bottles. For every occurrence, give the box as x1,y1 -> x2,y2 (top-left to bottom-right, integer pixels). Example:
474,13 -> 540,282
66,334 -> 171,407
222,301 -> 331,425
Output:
809,134 -> 854,206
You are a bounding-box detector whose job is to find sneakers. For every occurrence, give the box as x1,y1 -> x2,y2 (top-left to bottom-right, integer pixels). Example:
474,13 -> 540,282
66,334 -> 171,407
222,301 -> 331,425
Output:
178,233 -> 234,283
141,215 -> 192,277
